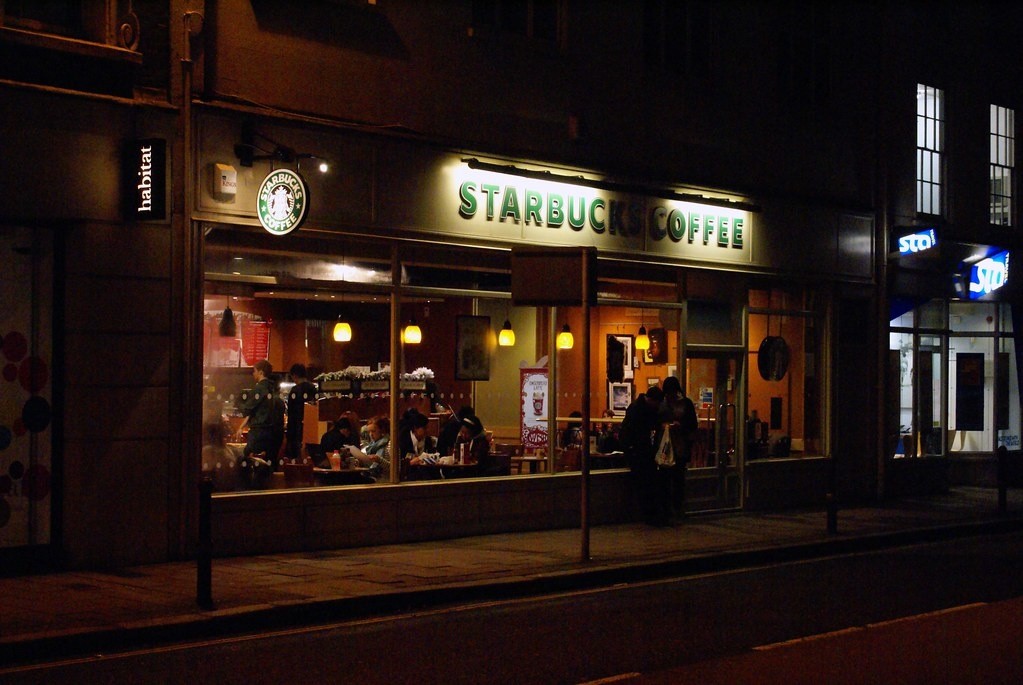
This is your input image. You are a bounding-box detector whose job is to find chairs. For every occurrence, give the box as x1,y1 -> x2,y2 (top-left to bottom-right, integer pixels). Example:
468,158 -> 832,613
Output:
201,428 -> 626,493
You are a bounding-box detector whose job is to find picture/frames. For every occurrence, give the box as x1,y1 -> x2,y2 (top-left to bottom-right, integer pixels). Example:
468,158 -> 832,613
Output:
607,332 -> 634,371
643,327 -> 669,365
455,314 -> 491,381
666,362 -> 677,378
647,376 -> 661,392
607,378 -> 634,416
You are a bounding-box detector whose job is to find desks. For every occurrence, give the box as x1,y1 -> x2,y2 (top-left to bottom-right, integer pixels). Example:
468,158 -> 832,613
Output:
588,451 -> 625,471
313,465 -> 371,486
509,455 -> 560,475
415,456 -> 479,481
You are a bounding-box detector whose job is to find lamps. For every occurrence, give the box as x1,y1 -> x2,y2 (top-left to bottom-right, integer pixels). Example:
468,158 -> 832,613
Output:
403,292 -> 422,344
218,230 -> 236,337
634,277 -> 650,349
758,289 -> 790,382
498,299 -> 515,347
333,254 -> 352,342
557,305 -> 574,349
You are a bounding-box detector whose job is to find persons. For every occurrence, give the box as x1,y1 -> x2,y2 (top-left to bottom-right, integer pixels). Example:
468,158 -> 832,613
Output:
319,416 -> 401,486
237,360 -> 286,472
284,363 -> 320,459
397,407 -> 443,482
596,410 -> 620,453
561,412 -> 583,449
201,422 -> 266,491
437,405 -> 491,479
617,376 -> 698,528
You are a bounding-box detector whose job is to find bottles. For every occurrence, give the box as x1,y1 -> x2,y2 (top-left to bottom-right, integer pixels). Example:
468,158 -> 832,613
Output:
452,443 -> 459,464
332,450 -> 340,470
435,450 -> 440,462
426,449 -> 428,453
350,457 -> 359,469
340,448 -> 350,469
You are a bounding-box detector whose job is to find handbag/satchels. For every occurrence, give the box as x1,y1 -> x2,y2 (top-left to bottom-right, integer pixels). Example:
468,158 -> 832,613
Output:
654,424 -> 677,470
564,433 -> 582,466
543,431 -> 565,464
254,381 -> 285,428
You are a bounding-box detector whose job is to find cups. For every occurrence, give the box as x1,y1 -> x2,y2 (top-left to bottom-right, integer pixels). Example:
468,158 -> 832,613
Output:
532,399 -> 543,415
431,438 -> 438,447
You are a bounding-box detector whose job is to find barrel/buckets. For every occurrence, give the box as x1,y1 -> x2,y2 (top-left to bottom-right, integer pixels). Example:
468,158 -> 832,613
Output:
284,463 -> 314,488
